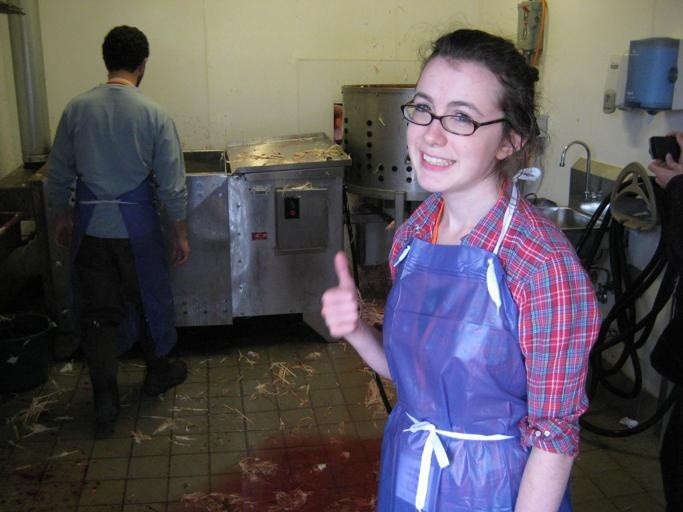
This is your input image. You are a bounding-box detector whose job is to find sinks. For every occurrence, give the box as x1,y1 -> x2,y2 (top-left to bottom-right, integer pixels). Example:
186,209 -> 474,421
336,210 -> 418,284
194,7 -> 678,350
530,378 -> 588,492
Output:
537,206 -> 611,250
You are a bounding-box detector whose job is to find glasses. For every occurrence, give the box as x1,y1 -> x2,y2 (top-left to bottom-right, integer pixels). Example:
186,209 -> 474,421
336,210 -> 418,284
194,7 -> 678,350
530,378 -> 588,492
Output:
400,97 -> 508,135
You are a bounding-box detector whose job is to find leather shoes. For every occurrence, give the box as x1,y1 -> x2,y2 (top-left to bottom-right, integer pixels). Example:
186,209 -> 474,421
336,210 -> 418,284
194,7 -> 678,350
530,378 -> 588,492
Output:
142,358 -> 189,397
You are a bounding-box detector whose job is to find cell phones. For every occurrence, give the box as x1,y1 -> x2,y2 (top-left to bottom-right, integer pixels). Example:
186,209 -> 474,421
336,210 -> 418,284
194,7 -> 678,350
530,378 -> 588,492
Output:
650,136 -> 682,161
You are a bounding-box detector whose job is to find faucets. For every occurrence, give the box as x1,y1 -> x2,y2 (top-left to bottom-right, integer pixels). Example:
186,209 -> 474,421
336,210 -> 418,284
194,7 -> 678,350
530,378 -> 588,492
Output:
560,140 -> 602,203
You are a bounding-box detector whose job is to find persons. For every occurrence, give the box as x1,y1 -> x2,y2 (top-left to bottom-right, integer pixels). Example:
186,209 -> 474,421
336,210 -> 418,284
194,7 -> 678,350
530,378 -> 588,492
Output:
648,131 -> 683,512
321,29 -> 601,512
45,25 -> 191,427
